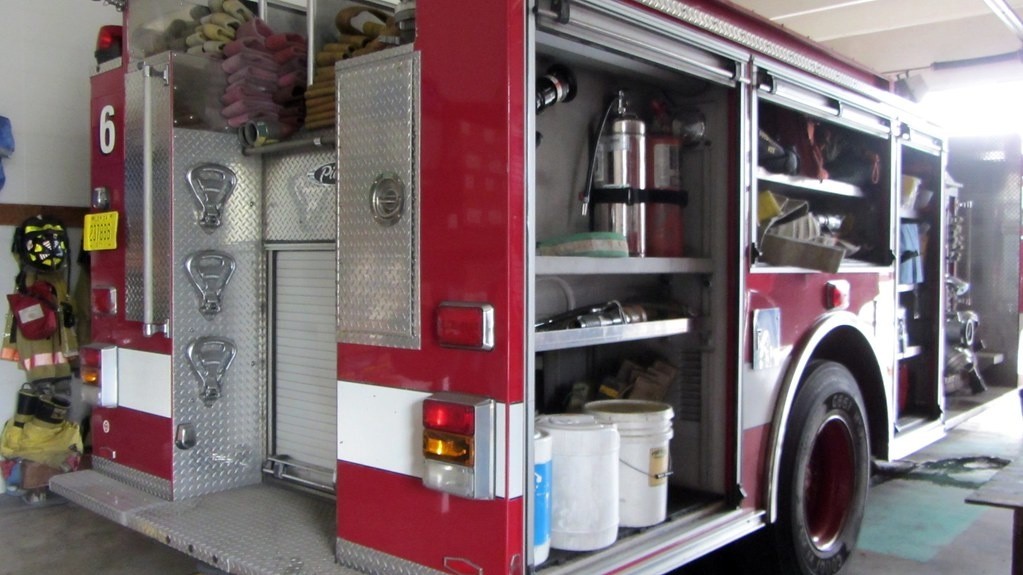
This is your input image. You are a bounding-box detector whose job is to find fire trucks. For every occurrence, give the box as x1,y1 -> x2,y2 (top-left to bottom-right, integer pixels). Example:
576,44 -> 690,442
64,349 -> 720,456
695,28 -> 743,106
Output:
47,0 -> 1023,575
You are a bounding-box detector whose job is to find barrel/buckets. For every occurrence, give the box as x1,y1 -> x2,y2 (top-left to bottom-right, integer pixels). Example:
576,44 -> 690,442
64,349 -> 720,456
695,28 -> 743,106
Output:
535,414 -> 621,551
535,428 -> 553,566
584,399 -> 674,528
